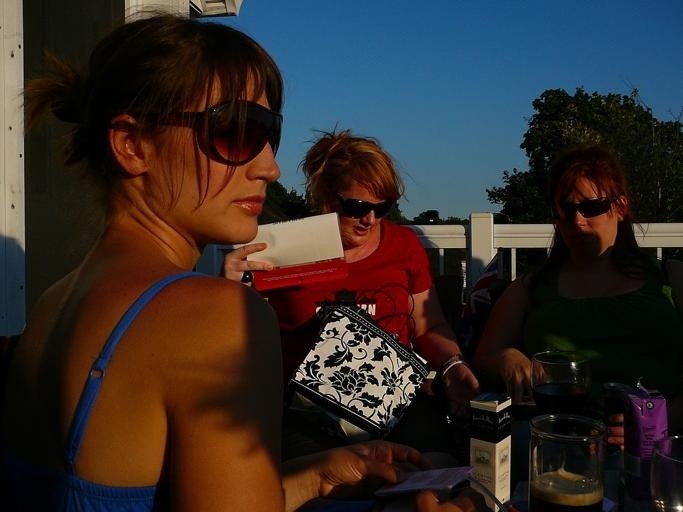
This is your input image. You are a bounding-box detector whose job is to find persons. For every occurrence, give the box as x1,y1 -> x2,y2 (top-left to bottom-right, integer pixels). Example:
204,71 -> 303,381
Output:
0,8 -> 486,510
473,140 -> 679,454
215,117 -> 487,448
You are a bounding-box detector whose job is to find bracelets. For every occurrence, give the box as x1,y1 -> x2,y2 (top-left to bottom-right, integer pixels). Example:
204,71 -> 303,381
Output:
437,353 -> 468,378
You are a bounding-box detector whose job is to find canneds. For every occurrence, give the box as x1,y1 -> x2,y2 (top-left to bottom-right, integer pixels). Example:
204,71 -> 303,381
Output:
597,383 -> 632,453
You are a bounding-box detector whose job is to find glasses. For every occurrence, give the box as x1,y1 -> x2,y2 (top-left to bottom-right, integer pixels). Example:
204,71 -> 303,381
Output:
332,193 -> 396,218
147,99 -> 283,164
556,193 -> 620,224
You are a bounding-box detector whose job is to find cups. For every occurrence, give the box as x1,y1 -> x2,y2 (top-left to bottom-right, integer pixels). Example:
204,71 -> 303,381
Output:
526,413 -> 609,512
648,435 -> 683,512
529,350 -> 593,413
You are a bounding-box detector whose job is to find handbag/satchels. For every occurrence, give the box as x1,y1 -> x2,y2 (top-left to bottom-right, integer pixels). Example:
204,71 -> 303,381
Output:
285,302 -> 428,444
